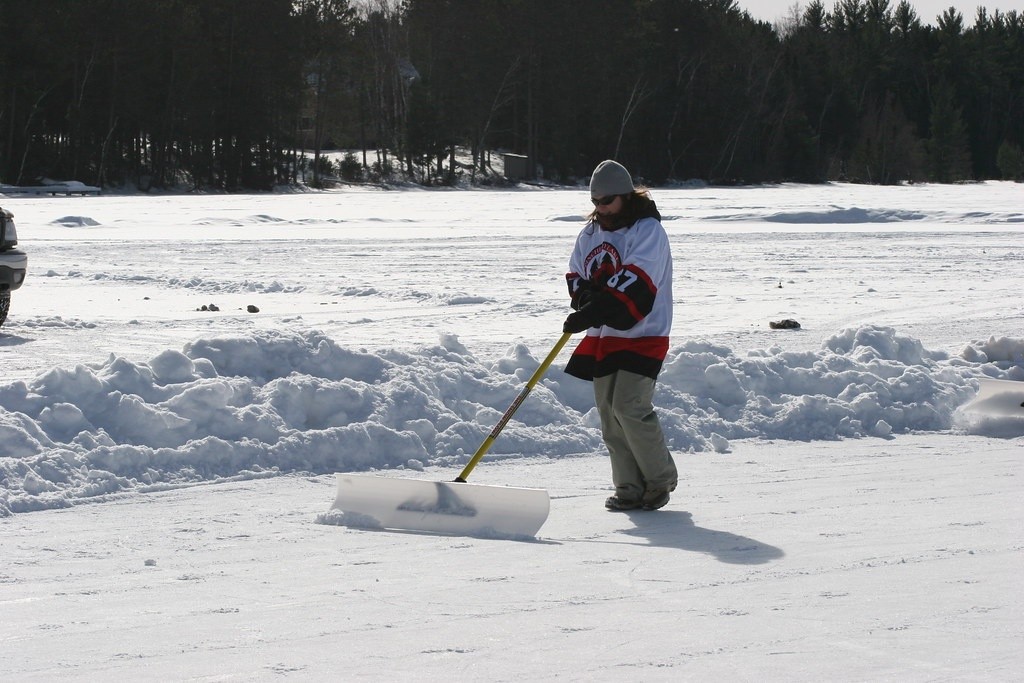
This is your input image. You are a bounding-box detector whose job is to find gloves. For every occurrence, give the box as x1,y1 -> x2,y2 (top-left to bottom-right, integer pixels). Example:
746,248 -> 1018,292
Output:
562,311 -> 593,333
578,290 -> 600,308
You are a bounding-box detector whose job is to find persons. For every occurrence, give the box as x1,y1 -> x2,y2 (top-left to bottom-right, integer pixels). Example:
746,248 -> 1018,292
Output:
561,158 -> 678,510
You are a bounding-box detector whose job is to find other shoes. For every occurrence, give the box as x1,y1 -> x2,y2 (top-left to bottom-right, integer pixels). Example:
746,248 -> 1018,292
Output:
641,486 -> 675,510
605,495 -> 642,510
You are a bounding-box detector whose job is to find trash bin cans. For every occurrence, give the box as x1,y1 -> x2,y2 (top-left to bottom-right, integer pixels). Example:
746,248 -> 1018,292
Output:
501,153 -> 528,186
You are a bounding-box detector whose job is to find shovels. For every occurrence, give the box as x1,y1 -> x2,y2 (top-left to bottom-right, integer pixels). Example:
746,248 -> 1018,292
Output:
328,309 -> 582,541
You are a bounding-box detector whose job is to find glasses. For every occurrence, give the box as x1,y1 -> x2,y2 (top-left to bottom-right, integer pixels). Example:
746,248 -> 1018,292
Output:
591,195 -> 618,207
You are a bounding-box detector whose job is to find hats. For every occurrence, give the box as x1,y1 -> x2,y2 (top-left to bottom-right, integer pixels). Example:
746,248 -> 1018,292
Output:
590,159 -> 634,198
